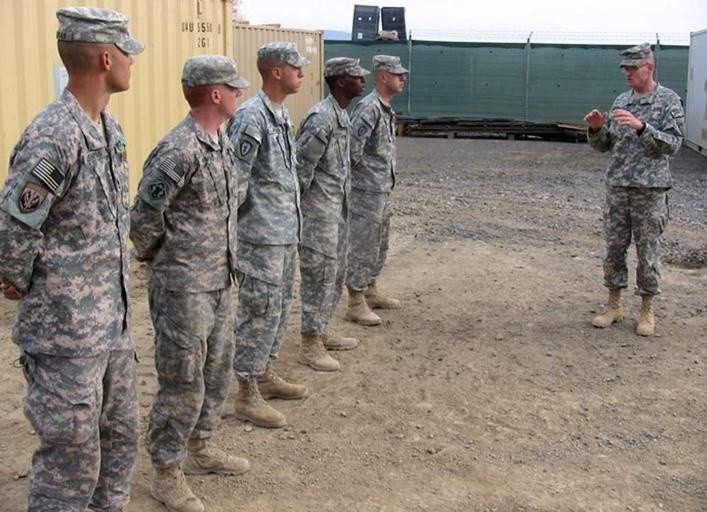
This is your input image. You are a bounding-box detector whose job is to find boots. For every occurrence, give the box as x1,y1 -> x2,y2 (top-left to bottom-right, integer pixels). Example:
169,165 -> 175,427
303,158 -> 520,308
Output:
232,378 -> 286,427
345,285 -> 381,326
180,438 -> 250,476
297,332 -> 341,371
255,366 -> 307,400
636,294 -> 655,335
590,289 -> 624,328
321,324 -> 357,350
365,277 -> 400,308
150,457 -> 205,512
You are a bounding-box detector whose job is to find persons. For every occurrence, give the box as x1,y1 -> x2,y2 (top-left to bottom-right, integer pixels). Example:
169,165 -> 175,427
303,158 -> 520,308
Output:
294,58 -> 370,375
0,7 -> 147,512
343,55 -> 408,326
225,39 -> 309,426
583,44 -> 686,336
129,54 -> 251,512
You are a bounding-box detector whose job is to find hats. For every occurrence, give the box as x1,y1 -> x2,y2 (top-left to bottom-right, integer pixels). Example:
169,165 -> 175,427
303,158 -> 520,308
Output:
618,42 -> 650,68
180,56 -> 249,90
56,6 -> 143,55
322,58 -> 371,77
256,42 -> 312,68
371,54 -> 409,76
